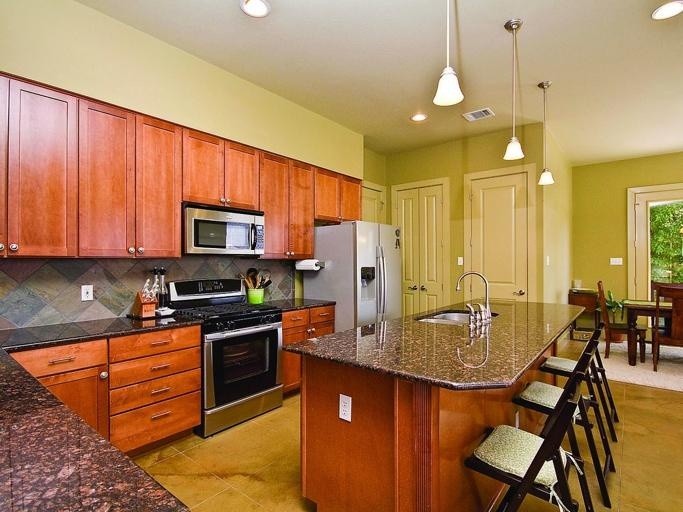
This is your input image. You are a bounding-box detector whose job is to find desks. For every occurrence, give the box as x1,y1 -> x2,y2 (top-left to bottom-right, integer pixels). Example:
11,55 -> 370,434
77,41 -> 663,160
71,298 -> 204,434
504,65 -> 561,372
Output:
623,299 -> 672,366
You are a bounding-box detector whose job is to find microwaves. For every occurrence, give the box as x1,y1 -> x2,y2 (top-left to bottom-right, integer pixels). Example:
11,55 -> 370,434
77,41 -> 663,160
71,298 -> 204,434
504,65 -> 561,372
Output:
180,201 -> 265,259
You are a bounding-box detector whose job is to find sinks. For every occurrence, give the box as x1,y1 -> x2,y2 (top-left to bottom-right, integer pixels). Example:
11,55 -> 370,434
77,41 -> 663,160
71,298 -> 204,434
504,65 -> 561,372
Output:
419,313 -> 469,327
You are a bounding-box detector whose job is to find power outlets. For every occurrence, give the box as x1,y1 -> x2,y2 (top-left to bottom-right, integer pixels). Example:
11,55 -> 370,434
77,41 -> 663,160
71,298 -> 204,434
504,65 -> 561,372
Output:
338,393 -> 352,423
80,285 -> 94,302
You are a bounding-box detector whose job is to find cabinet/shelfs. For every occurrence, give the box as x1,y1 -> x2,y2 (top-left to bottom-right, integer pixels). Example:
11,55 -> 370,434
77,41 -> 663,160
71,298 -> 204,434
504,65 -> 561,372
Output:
568,292 -> 599,341
282,306 -> 335,395
79,97 -> 183,259
108,324 -> 203,459
8,338 -> 110,444
260,151 -> 314,260
183,127 -> 259,212
0,76 -> 77,258
315,167 -> 361,222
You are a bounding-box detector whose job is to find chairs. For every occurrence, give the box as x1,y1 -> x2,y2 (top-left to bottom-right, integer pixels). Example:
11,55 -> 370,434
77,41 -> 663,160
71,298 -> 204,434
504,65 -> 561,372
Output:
464,371 -> 593,512
512,339 -> 615,508
652,283 -> 683,373
597,280 -> 648,363
539,322 -> 619,443
651,280 -> 683,329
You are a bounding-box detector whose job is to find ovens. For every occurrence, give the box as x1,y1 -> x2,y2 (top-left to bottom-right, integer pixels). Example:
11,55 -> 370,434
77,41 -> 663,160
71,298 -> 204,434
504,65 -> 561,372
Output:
192,322 -> 282,439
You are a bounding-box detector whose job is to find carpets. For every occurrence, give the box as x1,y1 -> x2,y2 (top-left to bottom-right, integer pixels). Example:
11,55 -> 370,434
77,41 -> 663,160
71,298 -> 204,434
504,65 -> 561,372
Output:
578,339 -> 683,392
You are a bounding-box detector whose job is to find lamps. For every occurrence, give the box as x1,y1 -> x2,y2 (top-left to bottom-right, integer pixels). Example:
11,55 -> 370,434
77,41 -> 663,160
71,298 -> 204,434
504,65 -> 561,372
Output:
432,0 -> 464,107
502,18 -> 526,161
537,80 -> 555,185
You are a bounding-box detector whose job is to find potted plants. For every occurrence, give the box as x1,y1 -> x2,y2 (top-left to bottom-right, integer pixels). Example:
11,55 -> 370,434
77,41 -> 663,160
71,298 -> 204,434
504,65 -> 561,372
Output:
595,291 -> 625,343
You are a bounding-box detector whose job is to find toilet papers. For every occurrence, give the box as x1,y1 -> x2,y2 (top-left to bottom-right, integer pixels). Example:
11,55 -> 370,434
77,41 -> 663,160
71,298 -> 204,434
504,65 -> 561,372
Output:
296,258 -> 320,271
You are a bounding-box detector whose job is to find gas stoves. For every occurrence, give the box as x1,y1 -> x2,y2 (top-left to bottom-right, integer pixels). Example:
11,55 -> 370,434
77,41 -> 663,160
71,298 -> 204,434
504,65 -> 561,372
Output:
166,277 -> 282,333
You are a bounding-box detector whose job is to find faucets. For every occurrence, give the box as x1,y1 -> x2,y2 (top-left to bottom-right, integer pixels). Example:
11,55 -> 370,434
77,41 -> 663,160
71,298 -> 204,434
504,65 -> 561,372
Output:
456,272 -> 491,321
456,321 -> 488,369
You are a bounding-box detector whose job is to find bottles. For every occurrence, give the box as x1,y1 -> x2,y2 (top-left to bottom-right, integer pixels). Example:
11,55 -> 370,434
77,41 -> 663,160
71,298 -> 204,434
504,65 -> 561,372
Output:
150,266 -> 161,294
159,267 -> 169,307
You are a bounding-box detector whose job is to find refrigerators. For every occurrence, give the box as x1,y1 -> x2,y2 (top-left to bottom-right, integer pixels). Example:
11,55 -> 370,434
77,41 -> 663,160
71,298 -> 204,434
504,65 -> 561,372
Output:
301,220 -> 400,332
